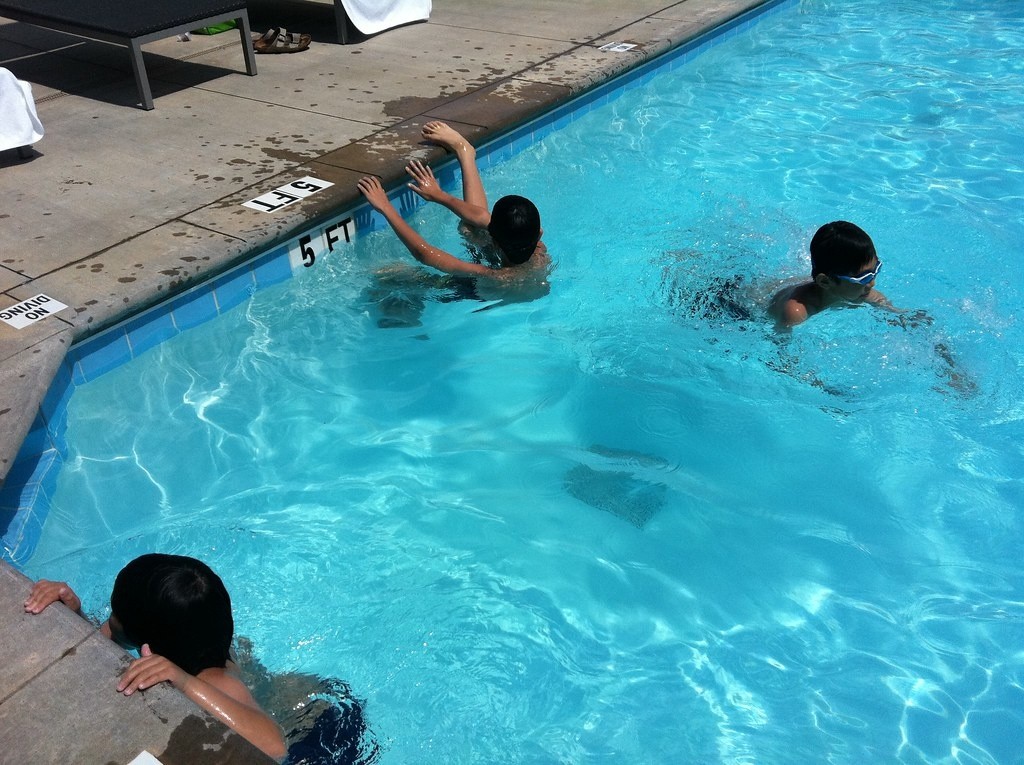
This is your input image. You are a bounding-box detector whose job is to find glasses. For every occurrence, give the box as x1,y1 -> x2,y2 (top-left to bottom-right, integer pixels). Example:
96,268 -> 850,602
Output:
835,262 -> 883,287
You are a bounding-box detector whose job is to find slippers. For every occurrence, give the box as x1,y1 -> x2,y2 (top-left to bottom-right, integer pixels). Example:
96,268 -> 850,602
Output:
255,27 -> 311,53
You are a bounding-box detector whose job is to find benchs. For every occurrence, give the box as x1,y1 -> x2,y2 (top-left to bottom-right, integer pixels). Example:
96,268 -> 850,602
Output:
0,0 -> 259,110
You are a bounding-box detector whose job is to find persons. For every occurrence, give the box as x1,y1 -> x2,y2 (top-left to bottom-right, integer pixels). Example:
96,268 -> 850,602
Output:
21,555 -> 287,765
356,122 -> 544,279
780,222 -> 883,325
